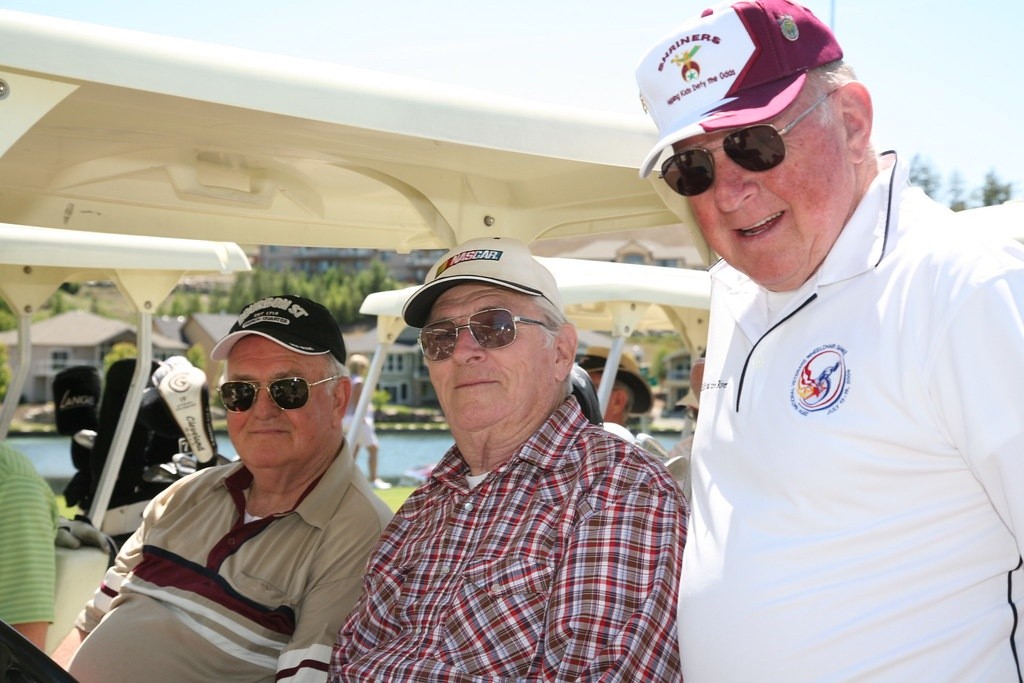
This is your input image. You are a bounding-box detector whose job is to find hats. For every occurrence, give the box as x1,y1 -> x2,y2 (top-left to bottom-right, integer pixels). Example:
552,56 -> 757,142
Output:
211,294 -> 347,365
675,364 -> 706,413
634,0 -> 844,178
575,345 -> 653,414
401,238 -> 569,330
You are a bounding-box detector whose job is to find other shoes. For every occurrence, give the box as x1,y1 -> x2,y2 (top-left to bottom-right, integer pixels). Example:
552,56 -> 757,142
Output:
366,478 -> 393,492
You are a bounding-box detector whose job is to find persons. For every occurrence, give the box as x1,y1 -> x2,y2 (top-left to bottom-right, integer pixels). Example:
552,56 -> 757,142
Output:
47,296 -> 393,683
0,443 -> 59,654
348,353 -> 391,490
328,236 -> 691,683
579,2 -> 1024,683
576,346 -> 654,430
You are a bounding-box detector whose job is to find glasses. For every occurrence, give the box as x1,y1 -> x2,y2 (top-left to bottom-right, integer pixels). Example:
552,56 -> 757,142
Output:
416,308 -> 553,362
216,372 -> 341,411
658,86 -> 839,196
